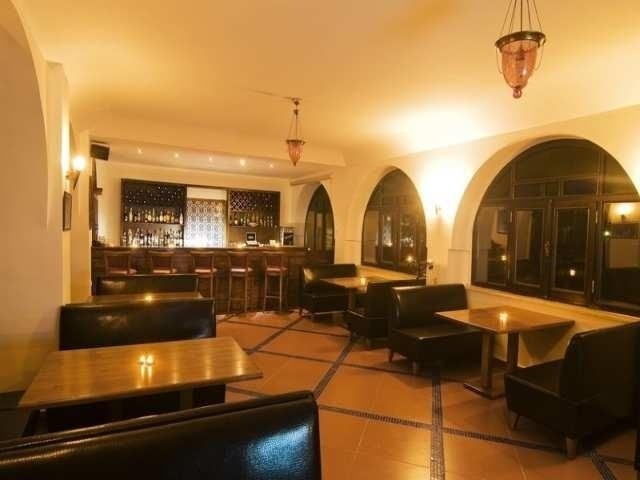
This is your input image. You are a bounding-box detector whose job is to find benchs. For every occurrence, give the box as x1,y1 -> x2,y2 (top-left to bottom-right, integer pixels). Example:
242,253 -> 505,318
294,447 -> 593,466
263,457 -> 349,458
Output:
58,298 -> 216,351
503,320 -> 640,459
344,279 -> 426,345
300,262 -> 359,318
0,390 -> 322,479
386,282 -> 485,375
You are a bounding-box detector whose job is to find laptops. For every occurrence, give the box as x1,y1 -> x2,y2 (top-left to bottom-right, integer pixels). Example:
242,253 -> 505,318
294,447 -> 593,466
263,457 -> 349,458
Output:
246,232 -> 259,246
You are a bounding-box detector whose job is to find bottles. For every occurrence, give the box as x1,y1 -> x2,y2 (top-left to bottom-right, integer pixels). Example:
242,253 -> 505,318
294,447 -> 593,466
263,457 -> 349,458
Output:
121,182 -> 185,248
230,190 -> 278,228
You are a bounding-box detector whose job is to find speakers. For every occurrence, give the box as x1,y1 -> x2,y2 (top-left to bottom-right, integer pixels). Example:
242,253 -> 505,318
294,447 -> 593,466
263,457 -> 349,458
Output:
90,143 -> 110,160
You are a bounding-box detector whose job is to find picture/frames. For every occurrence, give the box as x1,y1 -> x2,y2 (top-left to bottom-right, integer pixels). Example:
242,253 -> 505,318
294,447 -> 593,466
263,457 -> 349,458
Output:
62,190 -> 72,232
495,207 -> 511,234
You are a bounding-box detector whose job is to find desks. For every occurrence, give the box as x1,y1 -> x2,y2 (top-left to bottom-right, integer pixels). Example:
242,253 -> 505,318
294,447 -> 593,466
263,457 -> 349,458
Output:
319,272 -> 425,317
19,336 -> 263,431
433,304 -> 575,399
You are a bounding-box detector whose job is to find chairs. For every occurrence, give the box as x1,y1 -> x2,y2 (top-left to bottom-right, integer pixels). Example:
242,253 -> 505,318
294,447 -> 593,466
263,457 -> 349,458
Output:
101,249 -> 311,314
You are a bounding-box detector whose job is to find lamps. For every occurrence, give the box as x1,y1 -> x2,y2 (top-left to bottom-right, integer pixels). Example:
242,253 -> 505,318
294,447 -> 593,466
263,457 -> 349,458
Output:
493,0 -> 549,99
67,155 -> 87,190
283,92 -> 307,167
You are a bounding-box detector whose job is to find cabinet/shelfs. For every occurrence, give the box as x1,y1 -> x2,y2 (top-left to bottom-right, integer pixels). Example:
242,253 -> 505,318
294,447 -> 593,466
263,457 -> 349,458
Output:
119,177 -> 187,247
226,186 -> 281,247
